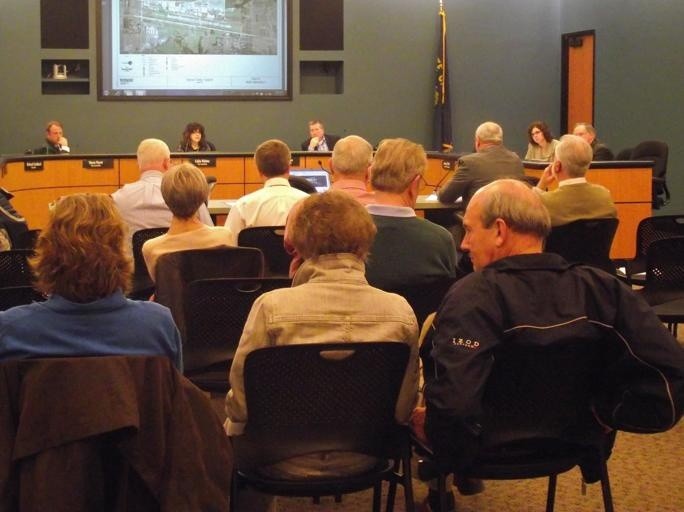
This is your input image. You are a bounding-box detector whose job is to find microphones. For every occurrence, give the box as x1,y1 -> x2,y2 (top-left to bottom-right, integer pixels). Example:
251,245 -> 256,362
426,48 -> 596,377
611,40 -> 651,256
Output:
318,160 -> 330,174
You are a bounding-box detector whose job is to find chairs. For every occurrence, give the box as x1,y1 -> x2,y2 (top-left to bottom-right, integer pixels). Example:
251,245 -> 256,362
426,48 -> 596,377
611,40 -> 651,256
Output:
1,138 -> 684,511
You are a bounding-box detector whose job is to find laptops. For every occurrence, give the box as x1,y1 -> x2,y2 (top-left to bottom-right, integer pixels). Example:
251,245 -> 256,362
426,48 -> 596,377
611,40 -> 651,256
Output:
289,170 -> 331,193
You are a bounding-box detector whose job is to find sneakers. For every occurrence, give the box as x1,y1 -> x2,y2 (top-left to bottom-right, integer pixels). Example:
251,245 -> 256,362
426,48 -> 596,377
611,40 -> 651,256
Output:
452,472 -> 484,496
415,494 -> 456,512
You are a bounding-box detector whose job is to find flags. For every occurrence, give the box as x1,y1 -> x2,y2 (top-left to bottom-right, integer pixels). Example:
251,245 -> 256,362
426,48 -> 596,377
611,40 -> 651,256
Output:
433,9 -> 454,151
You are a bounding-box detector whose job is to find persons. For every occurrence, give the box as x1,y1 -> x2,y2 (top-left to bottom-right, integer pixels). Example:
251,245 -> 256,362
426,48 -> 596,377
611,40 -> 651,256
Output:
174,121 -> 214,152
141,162 -> 236,302
325,135 -> 374,209
417,179 -> 683,512
533,130 -> 618,228
0,191 -> 183,377
45,119 -> 70,155
524,119 -> 560,162
299,119 -> 342,151
572,120 -> 615,163
224,139 -> 311,232
220,188 -> 421,437
354,136 -> 458,336
112,137 -> 217,274
435,121 -> 526,276
